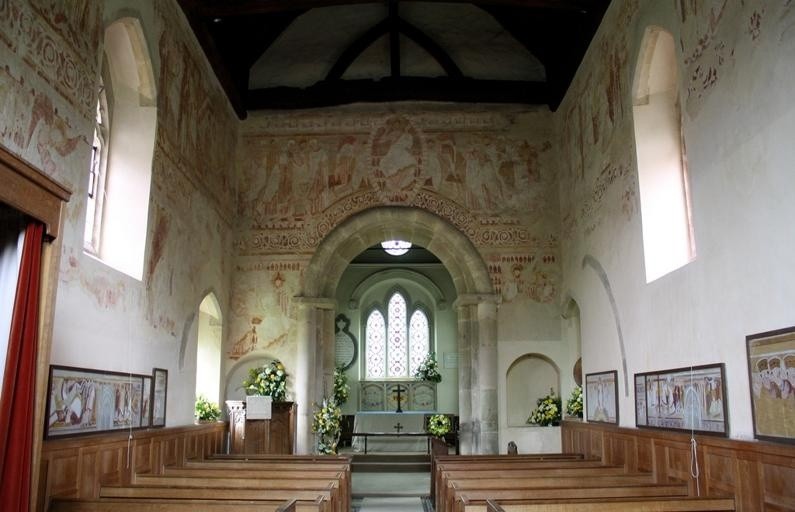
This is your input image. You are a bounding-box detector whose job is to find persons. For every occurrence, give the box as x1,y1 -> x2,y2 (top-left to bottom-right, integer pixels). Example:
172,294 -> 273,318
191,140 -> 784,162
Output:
639,376 -> 724,422
595,377 -> 609,422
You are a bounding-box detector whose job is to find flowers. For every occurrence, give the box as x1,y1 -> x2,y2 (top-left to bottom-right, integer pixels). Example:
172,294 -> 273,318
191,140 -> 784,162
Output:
526,385 -> 563,427
242,358 -> 290,402
312,396 -> 344,456
333,362 -> 352,405
413,351 -> 442,383
195,396 -> 221,425
566,388 -> 584,417
429,414 -> 450,436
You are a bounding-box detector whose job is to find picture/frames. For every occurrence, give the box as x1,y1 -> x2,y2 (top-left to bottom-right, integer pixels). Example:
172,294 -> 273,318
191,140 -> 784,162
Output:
153,367 -> 170,429
745,325 -> 795,444
585,370 -> 618,425
44,364 -> 155,441
633,372 -> 648,429
647,362 -> 729,438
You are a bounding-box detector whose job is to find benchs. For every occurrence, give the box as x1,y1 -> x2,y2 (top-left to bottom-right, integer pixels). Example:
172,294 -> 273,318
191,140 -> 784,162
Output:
428,451 -> 735,512
47,451 -> 352,512
